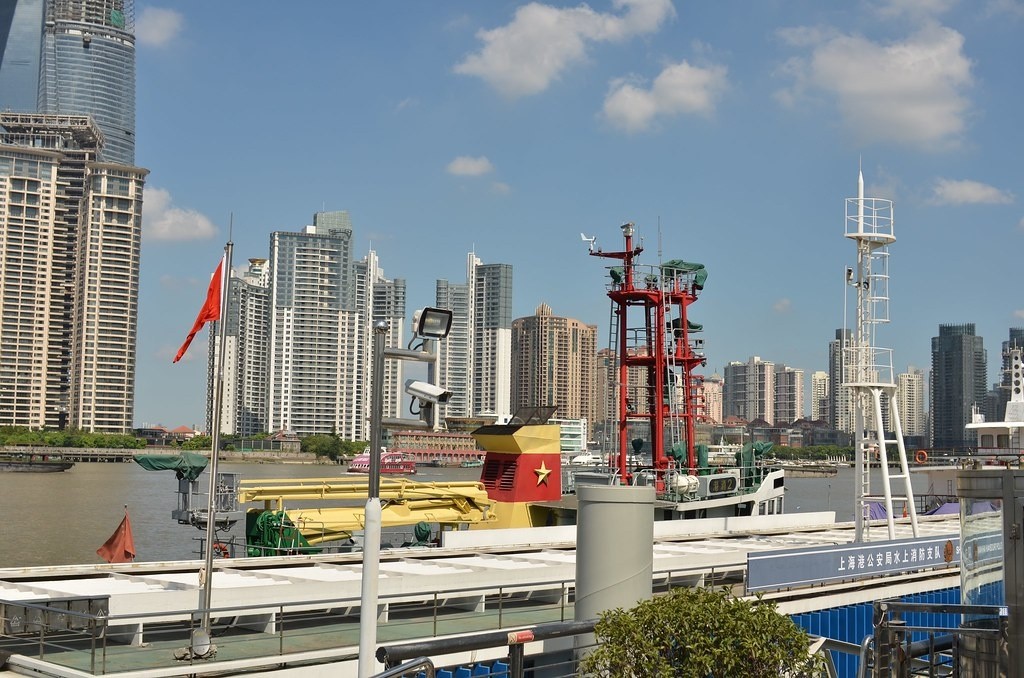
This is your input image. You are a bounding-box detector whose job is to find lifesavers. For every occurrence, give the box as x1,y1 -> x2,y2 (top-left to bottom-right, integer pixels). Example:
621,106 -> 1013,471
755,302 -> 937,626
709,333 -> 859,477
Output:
213,543 -> 229,559
916,450 -> 928,463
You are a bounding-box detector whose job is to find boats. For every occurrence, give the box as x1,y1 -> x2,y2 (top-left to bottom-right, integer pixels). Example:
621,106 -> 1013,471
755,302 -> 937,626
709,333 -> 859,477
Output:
346,444 -> 418,476
0,450 -> 77,474
458,459 -> 485,469
562,448 -> 854,494
132,220 -> 788,563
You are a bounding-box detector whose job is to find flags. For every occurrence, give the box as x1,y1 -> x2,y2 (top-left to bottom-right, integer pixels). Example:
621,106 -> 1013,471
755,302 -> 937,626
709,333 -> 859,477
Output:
173,253 -> 226,363
96,509 -> 135,563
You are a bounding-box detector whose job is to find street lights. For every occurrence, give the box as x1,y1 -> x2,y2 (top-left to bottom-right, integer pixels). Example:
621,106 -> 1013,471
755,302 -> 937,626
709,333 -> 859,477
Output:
358,307 -> 453,677
